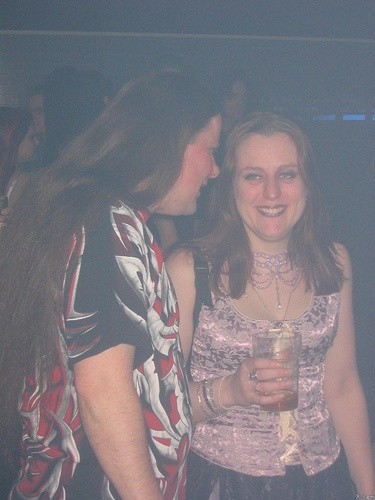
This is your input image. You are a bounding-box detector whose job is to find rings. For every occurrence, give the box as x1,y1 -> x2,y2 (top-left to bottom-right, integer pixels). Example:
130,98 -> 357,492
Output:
249,369 -> 257,380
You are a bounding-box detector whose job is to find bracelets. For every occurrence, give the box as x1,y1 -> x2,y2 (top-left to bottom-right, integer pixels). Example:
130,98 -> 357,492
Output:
197,375 -> 232,418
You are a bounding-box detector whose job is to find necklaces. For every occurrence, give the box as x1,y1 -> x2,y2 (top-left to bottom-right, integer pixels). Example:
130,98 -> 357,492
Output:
241,251 -> 305,321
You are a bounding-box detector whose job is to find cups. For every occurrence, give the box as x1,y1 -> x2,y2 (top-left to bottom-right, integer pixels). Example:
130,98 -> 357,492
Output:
254,331 -> 299,411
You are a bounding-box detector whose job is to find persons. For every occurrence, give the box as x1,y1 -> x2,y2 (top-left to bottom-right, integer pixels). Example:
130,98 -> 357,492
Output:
190,72 -> 246,238
0,71 -> 222,500
164,110 -> 375,500
0,84 -> 115,222
